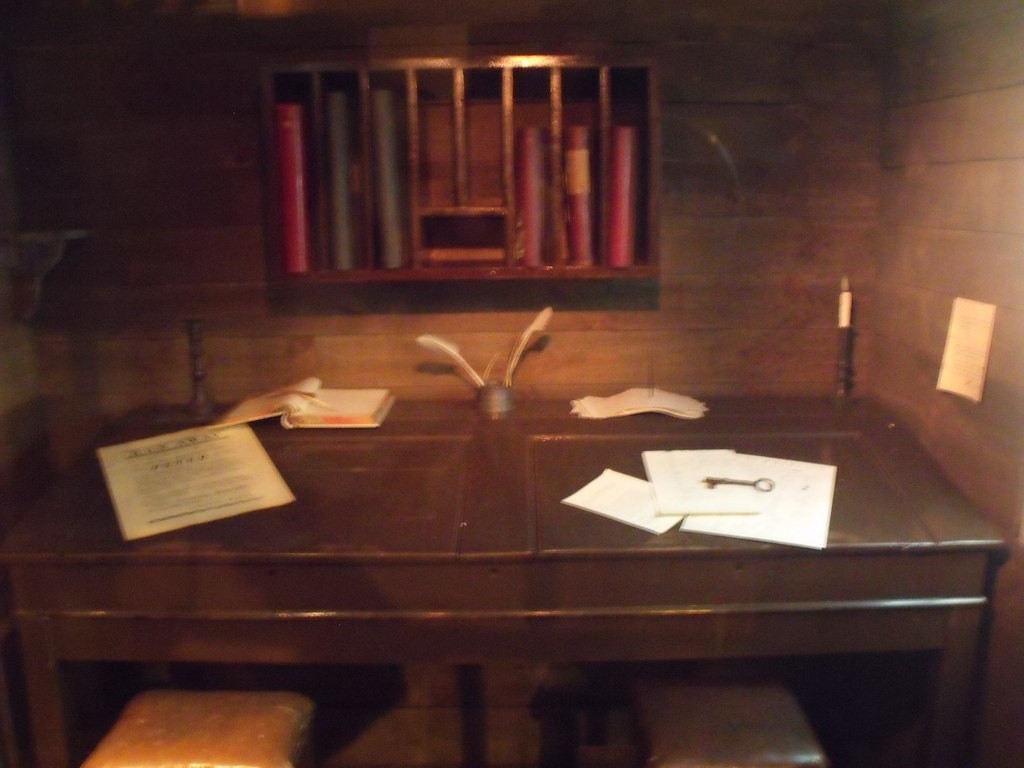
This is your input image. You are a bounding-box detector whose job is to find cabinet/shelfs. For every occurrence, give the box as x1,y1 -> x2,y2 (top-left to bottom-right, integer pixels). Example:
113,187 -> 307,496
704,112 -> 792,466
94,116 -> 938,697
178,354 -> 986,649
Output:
260,59 -> 664,284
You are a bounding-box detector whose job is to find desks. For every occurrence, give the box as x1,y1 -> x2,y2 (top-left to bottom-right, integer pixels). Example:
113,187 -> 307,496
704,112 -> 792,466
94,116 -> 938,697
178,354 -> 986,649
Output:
3,386 -> 1024,765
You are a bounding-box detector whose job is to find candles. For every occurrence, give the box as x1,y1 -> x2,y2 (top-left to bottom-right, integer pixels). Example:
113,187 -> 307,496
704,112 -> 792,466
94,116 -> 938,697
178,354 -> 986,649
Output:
838,285 -> 856,329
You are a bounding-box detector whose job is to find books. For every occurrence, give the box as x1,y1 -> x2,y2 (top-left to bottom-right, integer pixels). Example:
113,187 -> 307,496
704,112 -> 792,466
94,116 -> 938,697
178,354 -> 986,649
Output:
217,376 -> 398,431
326,94 -> 358,271
370,89 -> 409,269
561,126 -> 598,266
608,125 -> 640,270
276,103 -> 314,272
514,126 -> 555,264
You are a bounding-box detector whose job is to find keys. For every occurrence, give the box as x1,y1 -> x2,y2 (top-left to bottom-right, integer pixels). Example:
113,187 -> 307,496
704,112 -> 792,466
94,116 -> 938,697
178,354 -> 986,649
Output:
703,474 -> 776,496
